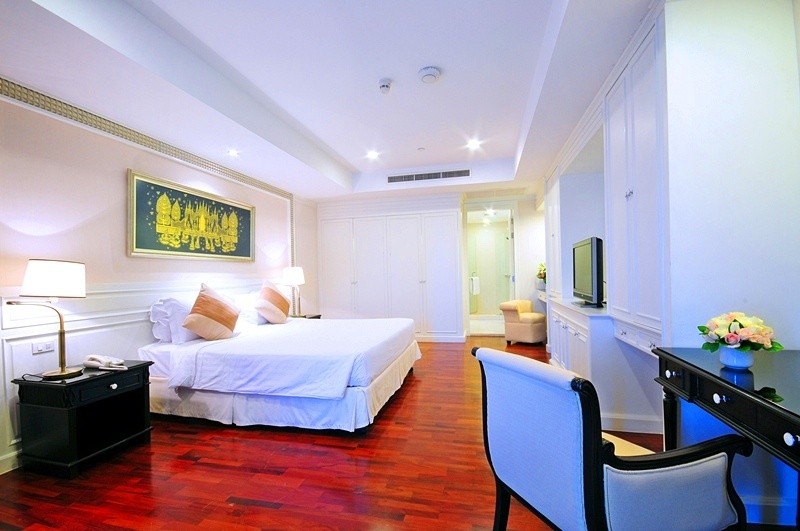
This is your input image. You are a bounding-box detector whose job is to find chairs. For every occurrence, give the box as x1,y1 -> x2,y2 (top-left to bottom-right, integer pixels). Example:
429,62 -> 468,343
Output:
471,347 -> 754,531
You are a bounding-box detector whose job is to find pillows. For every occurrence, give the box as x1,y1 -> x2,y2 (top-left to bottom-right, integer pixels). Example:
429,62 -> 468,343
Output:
149,280 -> 291,344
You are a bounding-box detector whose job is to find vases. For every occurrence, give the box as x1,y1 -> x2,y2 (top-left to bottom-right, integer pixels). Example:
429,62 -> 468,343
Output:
720,367 -> 754,390
719,345 -> 754,369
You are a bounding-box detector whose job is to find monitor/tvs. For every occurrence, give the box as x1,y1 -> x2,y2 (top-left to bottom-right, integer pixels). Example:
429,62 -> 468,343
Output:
572,237 -> 604,308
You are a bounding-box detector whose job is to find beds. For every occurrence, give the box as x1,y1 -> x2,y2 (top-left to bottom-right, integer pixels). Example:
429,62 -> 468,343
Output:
138,318 -> 422,434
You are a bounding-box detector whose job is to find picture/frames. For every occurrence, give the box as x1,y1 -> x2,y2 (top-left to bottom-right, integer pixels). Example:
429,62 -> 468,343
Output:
127,168 -> 256,264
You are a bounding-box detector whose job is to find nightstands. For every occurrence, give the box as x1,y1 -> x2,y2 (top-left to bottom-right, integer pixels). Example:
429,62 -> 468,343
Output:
11,360 -> 155,480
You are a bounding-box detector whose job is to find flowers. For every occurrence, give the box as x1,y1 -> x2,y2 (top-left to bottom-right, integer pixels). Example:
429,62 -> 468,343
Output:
697,312 -> 784,354
537,263 -> 546,284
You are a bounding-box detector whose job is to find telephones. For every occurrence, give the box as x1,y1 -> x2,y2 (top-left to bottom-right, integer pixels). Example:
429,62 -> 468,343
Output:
82,354 -> 124,367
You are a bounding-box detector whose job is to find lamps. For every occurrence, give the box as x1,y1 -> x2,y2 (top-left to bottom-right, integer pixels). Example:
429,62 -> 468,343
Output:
6,259 -> 86,380
282,267 -> 306,318
419,66 -> 440,84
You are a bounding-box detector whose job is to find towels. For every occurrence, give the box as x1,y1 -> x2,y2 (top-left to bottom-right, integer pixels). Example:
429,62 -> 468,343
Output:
470,277 -> 480,295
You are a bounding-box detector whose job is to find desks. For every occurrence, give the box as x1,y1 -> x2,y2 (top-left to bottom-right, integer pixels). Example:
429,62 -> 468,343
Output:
537,289 -> 546,303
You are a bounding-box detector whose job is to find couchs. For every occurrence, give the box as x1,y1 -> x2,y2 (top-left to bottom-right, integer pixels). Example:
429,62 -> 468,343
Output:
499,299 -> 546,346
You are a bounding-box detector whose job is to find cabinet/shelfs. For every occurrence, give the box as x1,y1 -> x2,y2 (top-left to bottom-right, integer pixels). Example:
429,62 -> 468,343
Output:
651,347 -> 800,531
546,24 -> 663,434
321,211 -> 466,343
